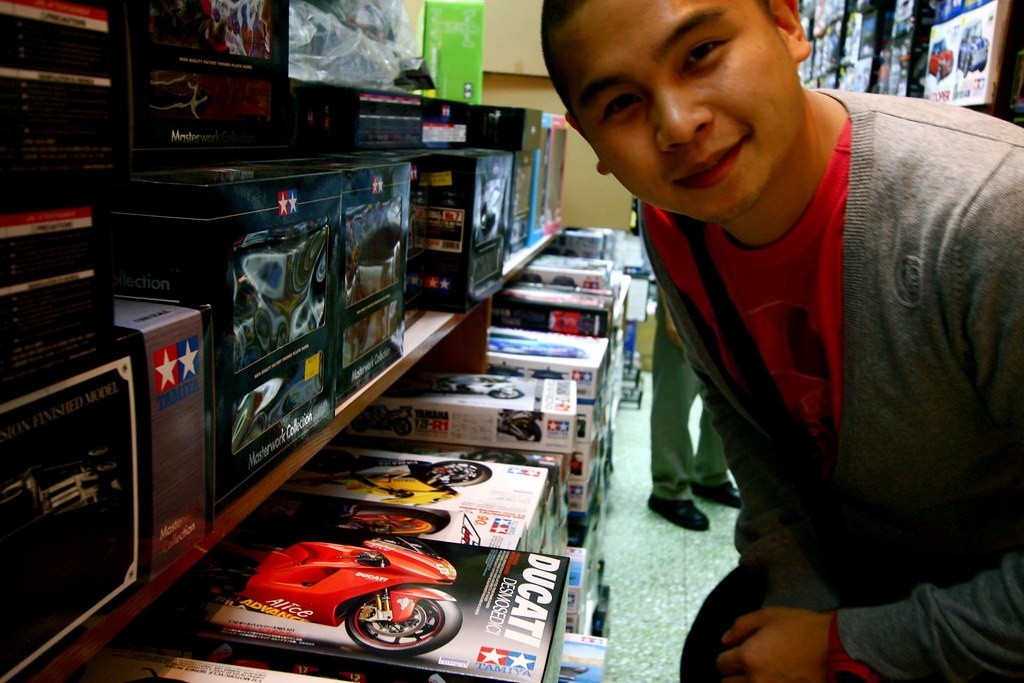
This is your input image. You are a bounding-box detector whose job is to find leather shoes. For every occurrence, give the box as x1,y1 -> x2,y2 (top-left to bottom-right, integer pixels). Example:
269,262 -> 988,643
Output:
648,493 -> 709,532
691,481 -> 742,508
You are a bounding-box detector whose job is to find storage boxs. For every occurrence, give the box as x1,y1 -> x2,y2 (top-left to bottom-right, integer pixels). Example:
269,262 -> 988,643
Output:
794,0 -> 1024,127
0,0 -> 651,683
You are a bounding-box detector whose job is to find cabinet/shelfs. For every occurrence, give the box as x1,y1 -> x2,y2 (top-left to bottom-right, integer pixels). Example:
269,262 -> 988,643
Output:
0,0 -> 630,683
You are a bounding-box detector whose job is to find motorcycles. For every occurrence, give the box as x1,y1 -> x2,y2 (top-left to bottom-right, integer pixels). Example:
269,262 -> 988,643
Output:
351,403 -> 414,435
184,502 -> 464,660
292,450 -> 494,506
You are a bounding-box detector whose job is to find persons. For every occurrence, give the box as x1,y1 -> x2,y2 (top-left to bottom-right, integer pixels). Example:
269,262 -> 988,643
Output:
647,283 -> 743,531
538,1 -> 1024,683
